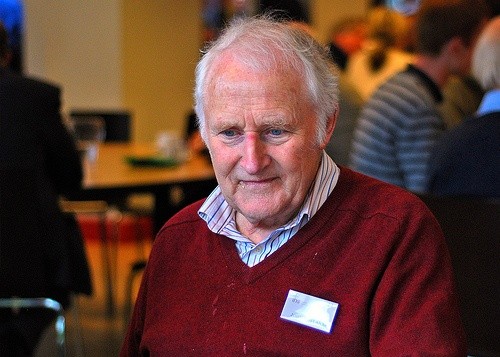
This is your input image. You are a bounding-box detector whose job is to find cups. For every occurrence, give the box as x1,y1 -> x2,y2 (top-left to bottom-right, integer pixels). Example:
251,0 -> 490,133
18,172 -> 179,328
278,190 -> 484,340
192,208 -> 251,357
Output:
67,116 -> 104,163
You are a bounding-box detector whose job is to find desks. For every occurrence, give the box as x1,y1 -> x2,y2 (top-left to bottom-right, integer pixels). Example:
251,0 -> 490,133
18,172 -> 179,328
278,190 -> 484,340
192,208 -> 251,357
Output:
65,141 -> 217,357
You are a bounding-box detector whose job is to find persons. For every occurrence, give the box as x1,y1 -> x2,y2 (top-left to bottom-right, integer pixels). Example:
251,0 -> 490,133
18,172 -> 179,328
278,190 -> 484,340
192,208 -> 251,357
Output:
151,1 -> 479,237
116,17 -> 471,357
428,15 -> 500,357
0,0 -> 98,357
348,0 -> 487,202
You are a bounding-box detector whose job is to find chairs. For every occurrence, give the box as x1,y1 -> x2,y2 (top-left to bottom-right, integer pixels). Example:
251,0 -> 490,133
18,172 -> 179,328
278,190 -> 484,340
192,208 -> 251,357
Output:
61,109 -> 132,322
